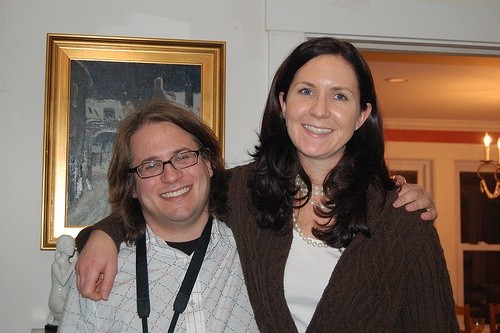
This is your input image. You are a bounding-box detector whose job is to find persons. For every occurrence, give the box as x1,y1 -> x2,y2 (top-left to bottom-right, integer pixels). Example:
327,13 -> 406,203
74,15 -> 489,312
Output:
57,100 -> 438,333
47,235 -> 75,325
75,37 -> 460,333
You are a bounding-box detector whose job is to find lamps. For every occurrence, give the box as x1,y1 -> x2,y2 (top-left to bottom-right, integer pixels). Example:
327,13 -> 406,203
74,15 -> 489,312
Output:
478,132 -> 500,198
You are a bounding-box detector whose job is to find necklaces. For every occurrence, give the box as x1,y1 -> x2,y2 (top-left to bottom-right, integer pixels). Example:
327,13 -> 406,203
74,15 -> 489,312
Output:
293,173 -> 336,248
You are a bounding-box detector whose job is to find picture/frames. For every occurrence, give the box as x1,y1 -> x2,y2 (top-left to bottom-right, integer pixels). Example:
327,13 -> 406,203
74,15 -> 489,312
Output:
41,33 -> 226,250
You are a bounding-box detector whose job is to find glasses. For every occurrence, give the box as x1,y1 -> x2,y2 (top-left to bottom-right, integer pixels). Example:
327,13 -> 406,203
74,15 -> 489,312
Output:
129,147 -> 209,180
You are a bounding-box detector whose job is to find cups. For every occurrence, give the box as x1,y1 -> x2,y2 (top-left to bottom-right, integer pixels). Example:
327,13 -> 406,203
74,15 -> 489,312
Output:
469,318 -> 485,333
